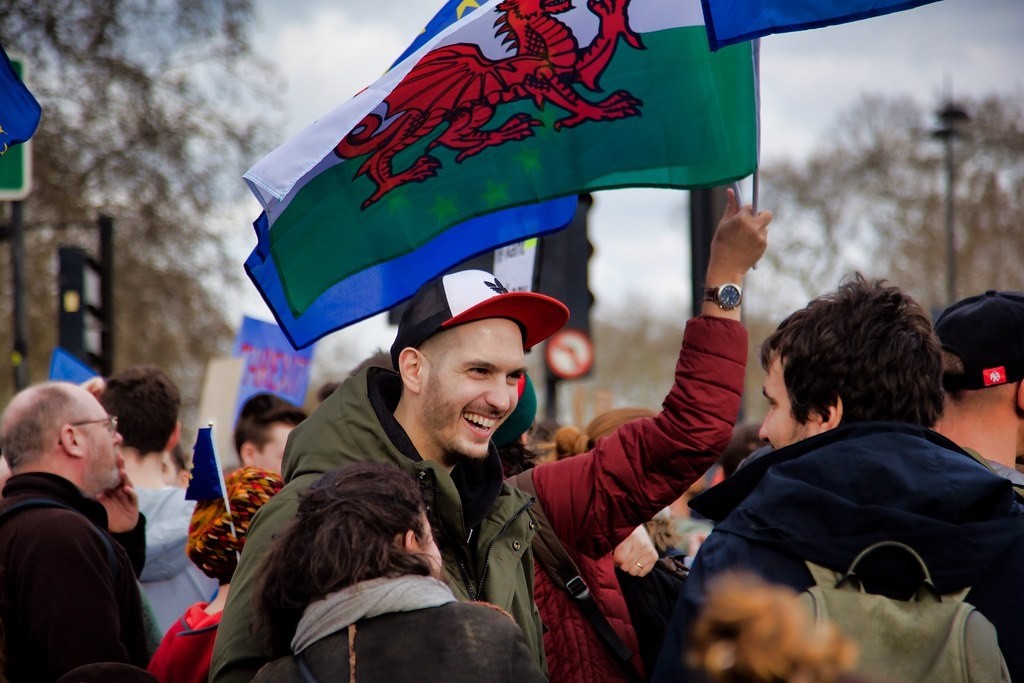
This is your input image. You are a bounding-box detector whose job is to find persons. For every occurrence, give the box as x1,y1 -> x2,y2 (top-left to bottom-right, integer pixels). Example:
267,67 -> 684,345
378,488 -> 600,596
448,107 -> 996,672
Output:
657,277 -> 1024,683
0,270 -> 1024,683
236,460 -> 550,683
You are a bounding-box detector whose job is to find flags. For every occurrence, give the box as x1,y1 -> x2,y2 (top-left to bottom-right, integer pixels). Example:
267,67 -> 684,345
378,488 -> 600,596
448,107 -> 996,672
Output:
244,0 -> 754,346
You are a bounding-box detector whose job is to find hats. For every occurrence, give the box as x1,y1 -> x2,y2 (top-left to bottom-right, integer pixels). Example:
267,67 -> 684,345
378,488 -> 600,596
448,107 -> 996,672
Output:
933,289 -> 1024,393
184,465 -> 285,578
390,269 -> 569,374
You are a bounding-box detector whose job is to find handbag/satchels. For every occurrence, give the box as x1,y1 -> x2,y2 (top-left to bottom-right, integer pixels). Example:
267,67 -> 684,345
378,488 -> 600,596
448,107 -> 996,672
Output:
796,541 -> 1012,683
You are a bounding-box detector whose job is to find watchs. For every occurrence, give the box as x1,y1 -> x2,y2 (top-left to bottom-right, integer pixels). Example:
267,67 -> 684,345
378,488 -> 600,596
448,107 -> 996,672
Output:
703,283 -> 744,310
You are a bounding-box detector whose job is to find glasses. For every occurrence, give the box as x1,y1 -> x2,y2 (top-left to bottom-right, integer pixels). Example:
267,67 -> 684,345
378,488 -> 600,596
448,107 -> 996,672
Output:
58,415 -> 118,446
